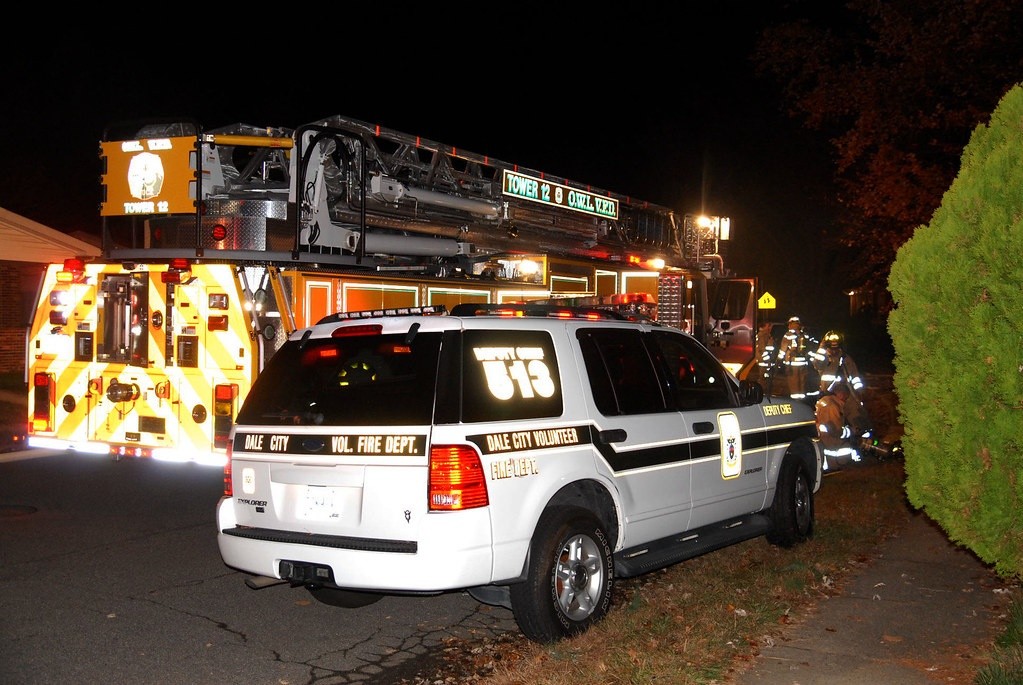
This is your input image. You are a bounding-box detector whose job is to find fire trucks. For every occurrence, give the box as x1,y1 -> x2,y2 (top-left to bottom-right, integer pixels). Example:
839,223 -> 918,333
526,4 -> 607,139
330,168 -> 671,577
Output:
8,113 -> 763,468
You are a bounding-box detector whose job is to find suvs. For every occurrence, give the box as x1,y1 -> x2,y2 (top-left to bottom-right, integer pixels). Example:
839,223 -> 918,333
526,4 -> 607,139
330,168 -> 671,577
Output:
217,290 -> 827,648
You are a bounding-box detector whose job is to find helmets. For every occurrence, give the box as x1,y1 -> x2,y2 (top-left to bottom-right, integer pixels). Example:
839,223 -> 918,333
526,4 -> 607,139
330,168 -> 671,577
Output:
825,331 -> 842,348
788,317 -> 801,328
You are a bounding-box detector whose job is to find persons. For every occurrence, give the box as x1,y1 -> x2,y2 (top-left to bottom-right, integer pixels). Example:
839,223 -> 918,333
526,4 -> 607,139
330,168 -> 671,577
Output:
817,385 -> 862,473
756,317 -> 862,431
